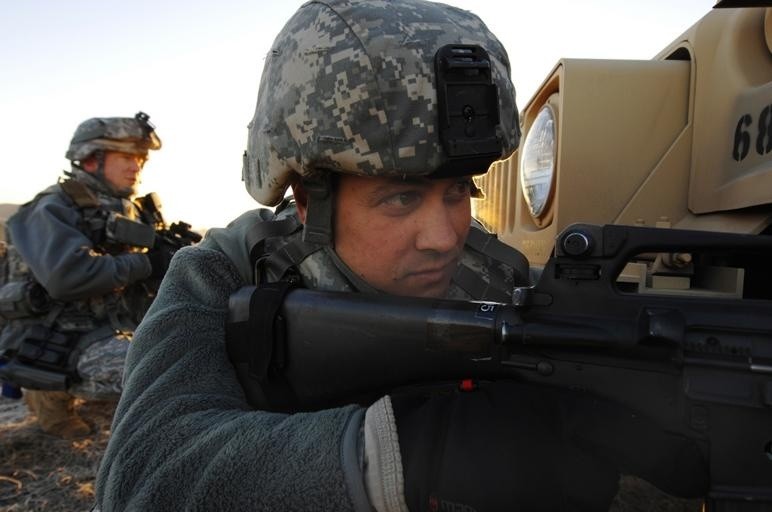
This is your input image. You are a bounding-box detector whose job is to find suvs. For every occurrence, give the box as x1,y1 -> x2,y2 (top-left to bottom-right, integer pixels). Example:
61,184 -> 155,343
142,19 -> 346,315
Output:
474,0 -> 772,298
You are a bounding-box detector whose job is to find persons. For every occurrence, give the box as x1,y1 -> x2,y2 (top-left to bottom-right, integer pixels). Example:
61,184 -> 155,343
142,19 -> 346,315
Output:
92,0 -> 711,512
0,116 -> 173,424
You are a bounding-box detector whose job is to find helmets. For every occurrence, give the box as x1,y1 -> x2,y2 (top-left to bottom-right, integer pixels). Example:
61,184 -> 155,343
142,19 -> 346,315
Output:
242,0 -> 522,207
66,111 -> 162,161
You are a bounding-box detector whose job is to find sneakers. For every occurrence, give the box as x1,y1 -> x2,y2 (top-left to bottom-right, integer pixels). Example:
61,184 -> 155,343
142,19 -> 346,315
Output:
19,388 -> 92,440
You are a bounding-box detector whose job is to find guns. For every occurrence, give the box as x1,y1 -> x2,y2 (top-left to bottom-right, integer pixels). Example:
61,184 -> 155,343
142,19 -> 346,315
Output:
115,216 -> 204,259
225,222 -> 772,512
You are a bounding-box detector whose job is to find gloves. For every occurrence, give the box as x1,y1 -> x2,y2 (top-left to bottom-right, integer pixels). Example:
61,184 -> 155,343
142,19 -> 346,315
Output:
145,248 -> 175,279
406,380 -> 711,512
